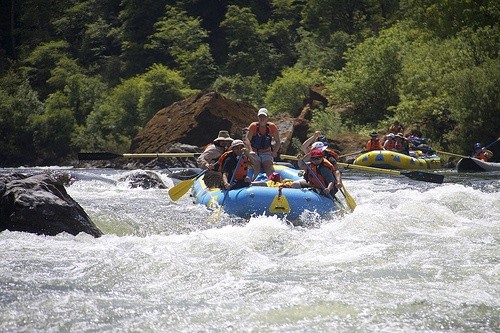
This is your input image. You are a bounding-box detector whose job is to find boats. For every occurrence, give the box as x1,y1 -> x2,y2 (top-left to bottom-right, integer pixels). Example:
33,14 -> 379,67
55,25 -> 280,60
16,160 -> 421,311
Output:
457,155 -> 500,172
352,149 -> 442,171
190,164 -> 336,226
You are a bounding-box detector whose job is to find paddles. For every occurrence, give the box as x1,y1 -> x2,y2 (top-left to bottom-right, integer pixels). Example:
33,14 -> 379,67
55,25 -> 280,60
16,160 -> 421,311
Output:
299,156 -> 348,217
336,150 -> 363,159
475,138 -> 500,158
168,162 -> 219,201
209,153 -> 244,221
279,154 -> 444,184
435,150 -> 468,158
76,153 -> 201,160
338,178 -> 357,213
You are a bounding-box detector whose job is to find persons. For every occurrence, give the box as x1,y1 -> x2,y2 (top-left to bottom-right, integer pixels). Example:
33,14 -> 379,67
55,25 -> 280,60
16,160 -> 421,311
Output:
366,131 -> 382,150
197,131 -> 233,188
383,121 -> 422,155
246,107 -> 281,182
291,130 -> 343,199
469,143 -> 493,162
222,139 -> 254,191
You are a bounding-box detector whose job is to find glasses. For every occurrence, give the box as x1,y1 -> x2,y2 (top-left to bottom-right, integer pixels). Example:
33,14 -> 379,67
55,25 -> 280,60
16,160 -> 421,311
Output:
311,156 -> 322,161
235,146 -> 243,149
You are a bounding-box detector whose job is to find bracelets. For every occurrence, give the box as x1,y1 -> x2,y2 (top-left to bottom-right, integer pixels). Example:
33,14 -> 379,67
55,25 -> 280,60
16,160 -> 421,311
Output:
337,180 -> 342,183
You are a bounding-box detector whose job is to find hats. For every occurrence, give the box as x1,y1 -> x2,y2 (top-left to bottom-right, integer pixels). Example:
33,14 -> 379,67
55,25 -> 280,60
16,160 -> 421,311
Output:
227,139 -> 244,150
370,133 -> 379,137
393,120 -> 399,124
257,108 -> 269,117
310,150 -> 323,157
214,130 -> 234,142
395,133 -> 404,139
311,142 -> 328,152
386,132 -> 395,139
473,142 -> 482,149
